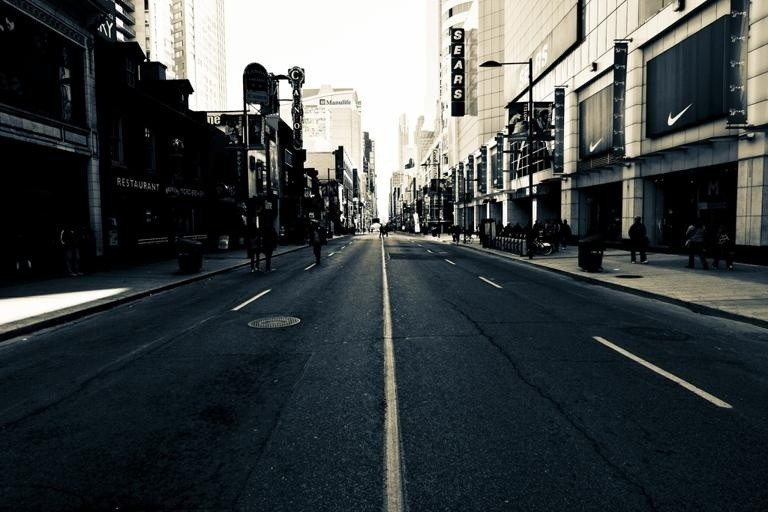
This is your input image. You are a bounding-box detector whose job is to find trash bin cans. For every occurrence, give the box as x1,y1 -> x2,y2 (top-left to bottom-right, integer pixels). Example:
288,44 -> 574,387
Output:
578,237 -> 604,269
177,238 -> 203,274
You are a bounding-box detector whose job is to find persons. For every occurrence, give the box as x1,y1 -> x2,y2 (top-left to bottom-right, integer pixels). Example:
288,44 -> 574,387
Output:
478,219 -> 570,259
311,225 -> 322,264
62,229 -> 84,276
263,234 -> 276,272
380,224 -> 383,238
628,217 -> 648,264
685,217 -> 736,271
248,234 -> 260,273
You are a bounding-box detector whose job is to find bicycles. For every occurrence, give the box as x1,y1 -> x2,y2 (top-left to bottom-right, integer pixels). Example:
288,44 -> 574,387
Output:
495,232 -> 554,258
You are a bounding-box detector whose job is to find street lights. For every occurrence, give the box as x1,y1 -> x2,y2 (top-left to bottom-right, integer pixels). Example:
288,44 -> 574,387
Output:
421,161 -> 443,238
480,57 -> 535,244
